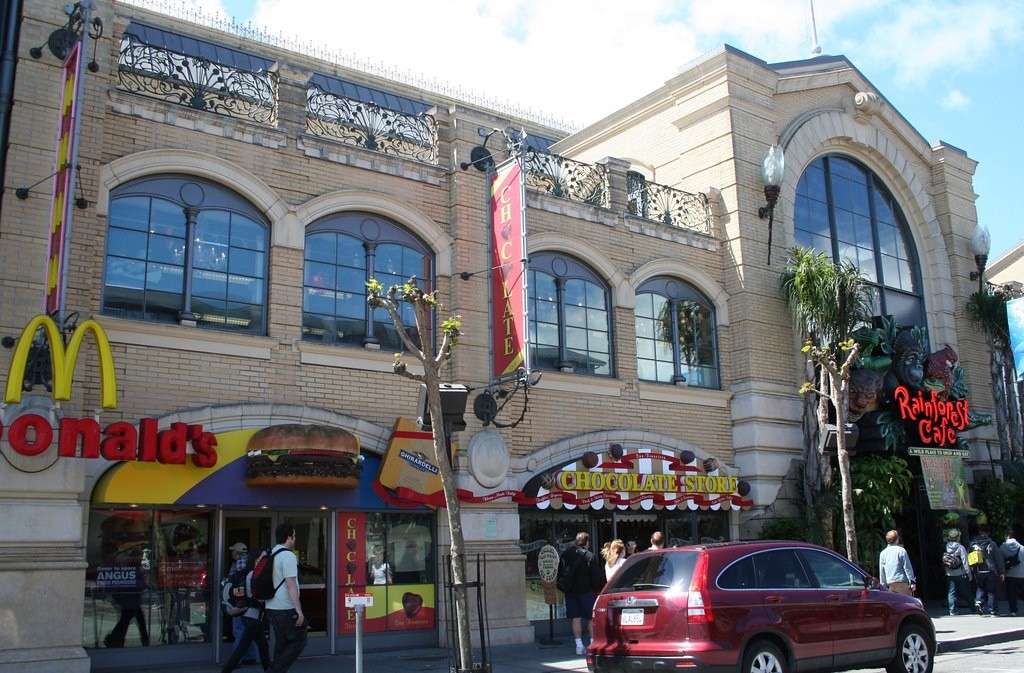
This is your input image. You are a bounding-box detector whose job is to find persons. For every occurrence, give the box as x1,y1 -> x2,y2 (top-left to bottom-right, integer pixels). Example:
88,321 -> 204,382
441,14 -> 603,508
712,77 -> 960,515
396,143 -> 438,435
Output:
879,529 -> 917,596
645,531 -> 664,552
999,528 -> 1024,617
624,539 -> 638,559
945,529 -> 974,615
969,523 -> 1005,618
222,523 -> 309,673
600,539 -> 626,585
103,549 -> 155,647
368,541 -> 420,585
559,531 -> 603,656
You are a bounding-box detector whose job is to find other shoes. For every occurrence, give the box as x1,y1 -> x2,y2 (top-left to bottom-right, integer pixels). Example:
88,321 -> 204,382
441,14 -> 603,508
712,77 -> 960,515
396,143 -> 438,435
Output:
950,609 -> 959,616
975,600 -> 983,614
222,659 -> 241,668
242,658 -> 256,665
1009,611 -> 1016,617
991,609 -> 999,615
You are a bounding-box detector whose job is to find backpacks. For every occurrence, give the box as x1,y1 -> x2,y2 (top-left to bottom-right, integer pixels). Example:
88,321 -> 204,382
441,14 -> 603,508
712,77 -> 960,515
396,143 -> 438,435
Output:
222,567 -> 255,617
251,548 -> 294,600
556,549 -> 588,594
942,543 -> 962,569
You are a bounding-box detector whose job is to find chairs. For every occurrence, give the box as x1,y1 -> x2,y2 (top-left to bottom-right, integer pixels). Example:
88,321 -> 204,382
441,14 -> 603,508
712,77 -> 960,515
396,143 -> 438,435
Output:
764,570 -> 788,587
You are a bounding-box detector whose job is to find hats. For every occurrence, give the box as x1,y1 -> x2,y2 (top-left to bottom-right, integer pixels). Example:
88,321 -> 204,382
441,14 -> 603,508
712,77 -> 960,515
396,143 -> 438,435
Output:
886,528 -> 899,543
229,542 -> 247,552
948,529 -> 961,540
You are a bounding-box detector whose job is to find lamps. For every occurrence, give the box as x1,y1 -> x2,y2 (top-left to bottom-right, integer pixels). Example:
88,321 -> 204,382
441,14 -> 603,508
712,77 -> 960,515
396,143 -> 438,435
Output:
758,143 -> 785,266
968,224 -> 992,315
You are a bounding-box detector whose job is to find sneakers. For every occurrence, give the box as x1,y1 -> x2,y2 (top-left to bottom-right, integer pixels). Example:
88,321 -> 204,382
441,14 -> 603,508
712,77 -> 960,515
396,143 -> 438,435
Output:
576,642 -> 587,655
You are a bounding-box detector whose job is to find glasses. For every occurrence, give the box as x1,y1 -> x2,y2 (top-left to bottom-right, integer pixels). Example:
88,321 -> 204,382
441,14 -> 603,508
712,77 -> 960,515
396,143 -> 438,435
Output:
627,544 -> 636,547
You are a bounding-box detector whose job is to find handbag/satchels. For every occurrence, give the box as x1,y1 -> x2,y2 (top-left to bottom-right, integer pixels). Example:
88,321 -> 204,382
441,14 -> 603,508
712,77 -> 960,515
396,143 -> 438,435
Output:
384,562 -> 392,584
1003,547 -> 1020,571
968,549 -> 983,566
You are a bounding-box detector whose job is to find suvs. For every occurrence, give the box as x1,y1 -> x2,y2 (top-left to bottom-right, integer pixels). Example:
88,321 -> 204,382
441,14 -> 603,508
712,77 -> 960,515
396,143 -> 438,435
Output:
584,540 -> 939,673
